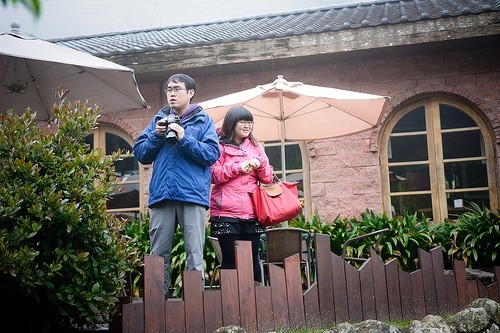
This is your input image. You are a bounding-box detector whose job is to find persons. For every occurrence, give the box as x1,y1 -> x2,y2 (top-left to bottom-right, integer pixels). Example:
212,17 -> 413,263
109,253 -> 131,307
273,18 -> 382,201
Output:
207,107 -> 273,285
135,74 -> 220,299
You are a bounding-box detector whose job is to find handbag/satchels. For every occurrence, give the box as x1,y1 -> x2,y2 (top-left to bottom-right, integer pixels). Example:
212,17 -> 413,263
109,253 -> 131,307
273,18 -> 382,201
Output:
250,162 -> 302,225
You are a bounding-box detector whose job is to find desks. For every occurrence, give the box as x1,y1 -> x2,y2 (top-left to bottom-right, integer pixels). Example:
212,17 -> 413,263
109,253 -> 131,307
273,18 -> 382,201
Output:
260,233 -> 330,283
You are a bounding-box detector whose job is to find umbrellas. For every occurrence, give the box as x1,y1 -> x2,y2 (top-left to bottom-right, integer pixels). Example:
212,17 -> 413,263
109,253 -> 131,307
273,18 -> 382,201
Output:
190,75 -> 391,229
0,22 -> 150,122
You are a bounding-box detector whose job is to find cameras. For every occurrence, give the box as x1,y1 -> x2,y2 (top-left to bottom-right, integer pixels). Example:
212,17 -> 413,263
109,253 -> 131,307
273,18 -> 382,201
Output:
160,114 -> 181,144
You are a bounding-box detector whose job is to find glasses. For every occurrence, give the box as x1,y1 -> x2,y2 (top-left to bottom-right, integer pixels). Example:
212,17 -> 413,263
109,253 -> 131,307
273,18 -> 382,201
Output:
238,120 -> 254,127
163,88 -> 186,95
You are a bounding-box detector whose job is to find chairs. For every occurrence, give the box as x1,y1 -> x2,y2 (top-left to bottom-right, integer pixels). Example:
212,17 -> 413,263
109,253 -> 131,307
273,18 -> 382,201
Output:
207,228 -> 390,290
